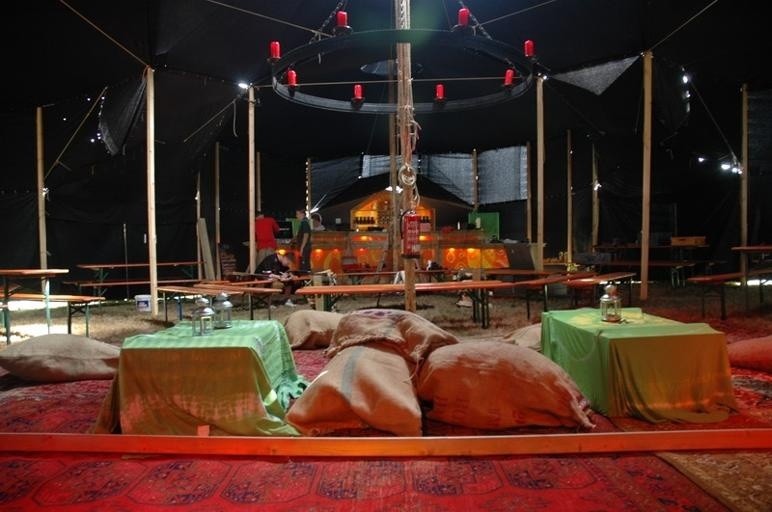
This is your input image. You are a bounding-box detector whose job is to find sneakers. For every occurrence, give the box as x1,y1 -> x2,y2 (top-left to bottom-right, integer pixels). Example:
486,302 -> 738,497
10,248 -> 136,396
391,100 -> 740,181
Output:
285,300 -> 295,306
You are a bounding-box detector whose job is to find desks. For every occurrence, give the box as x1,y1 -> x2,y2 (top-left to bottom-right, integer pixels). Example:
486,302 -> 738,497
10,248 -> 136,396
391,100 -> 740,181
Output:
85,319 -> 310,436
541,307 -> 739,424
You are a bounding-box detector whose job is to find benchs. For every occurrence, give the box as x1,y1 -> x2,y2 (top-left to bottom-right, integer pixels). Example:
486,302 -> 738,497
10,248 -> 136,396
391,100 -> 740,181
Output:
2,266 -> 69,279
2,290 -> 106,318
155,270 -> 638,296
685,271 -> 753,283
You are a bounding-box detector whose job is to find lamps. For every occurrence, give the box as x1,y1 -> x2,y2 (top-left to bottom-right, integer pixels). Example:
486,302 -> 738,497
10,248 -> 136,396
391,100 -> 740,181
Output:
269,0 -> 536,115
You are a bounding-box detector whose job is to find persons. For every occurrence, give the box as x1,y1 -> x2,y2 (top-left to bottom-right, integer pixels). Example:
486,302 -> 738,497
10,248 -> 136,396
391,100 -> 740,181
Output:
253,209 -> 281,268
294,205 -> 313,272
309,213 -> 327,232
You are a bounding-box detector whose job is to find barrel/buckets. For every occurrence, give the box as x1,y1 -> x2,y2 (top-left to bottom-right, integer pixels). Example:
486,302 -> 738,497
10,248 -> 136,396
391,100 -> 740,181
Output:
134,295 -> 151,312
